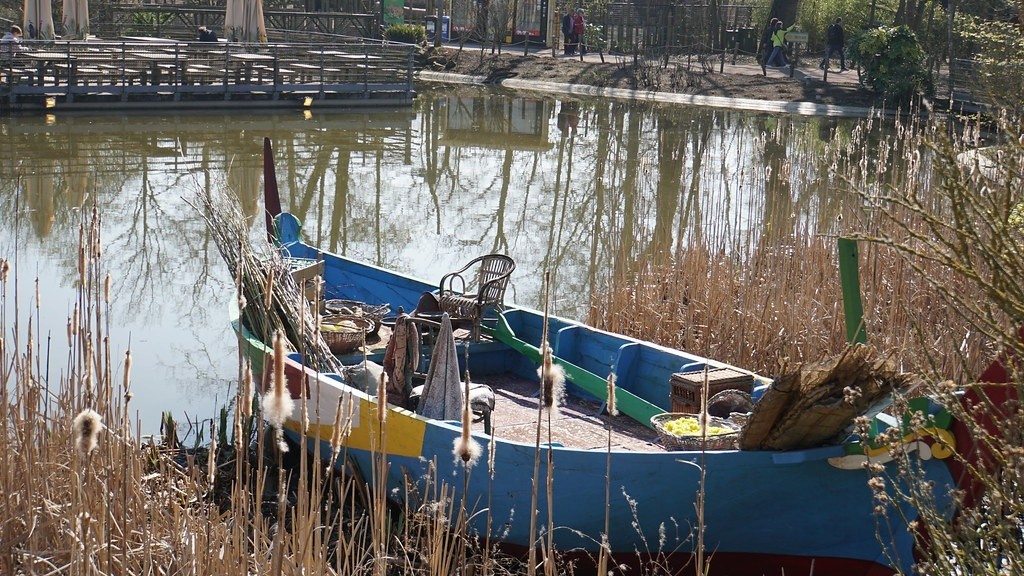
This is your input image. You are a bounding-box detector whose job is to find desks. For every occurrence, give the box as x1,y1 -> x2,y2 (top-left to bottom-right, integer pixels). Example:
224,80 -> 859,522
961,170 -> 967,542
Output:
230,54 -> 279,86
131,54 -> 188,86
335,55 -> 383,84
306,50 -> 350,81
22,53 -> 77,86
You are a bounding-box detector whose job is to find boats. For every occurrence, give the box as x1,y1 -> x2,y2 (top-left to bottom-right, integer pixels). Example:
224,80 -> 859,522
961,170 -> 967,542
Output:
231,137 -> 1024,576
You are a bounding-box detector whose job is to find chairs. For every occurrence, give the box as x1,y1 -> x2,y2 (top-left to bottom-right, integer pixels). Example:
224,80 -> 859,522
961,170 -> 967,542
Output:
440,254 -> 516,341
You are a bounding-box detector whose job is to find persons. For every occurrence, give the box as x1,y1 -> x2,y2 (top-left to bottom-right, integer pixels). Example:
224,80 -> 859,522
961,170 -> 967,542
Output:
820,18 -> 848,71
198,26 -> 220,47
763,18 -> 796,68
1,25 -> 32,68
562,8 -> 588,55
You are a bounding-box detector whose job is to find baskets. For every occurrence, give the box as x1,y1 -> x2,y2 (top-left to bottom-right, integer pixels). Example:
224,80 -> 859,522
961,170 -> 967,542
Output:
650,389 -> 754,452
320,314 -> 375,354
325,299 -> 391,335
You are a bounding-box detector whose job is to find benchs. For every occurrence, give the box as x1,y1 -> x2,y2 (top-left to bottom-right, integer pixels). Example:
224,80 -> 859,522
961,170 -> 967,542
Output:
3,36 -> 385,93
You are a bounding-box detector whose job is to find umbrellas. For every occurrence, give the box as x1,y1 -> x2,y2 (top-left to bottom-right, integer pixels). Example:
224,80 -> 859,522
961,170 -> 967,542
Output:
62,0 -> 90,40
224,0 -> 268,47
23,0 -> 55,48
415,313 -> 463,423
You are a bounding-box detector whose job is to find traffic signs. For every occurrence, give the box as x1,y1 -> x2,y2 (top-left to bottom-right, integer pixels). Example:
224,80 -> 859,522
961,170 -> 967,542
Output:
784,32 -> 809,42
